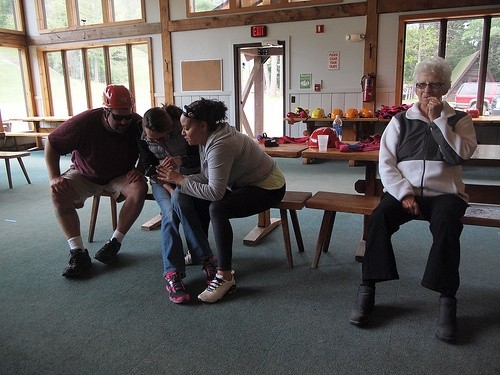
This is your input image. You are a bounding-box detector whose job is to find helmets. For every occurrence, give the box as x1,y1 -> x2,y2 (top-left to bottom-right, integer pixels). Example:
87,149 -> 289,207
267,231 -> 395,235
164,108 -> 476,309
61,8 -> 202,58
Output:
102,84 -> 135,109
309,127 -> 339,148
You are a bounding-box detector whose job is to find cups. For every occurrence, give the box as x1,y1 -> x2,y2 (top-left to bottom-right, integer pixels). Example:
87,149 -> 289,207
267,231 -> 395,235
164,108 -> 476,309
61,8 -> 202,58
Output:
317,135 -> 329,153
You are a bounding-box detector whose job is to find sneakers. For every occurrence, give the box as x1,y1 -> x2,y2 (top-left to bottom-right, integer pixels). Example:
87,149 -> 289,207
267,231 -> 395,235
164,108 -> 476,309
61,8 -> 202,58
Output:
197,272 -> 236,304
62,247 -> 92,277
184,250 -> 193,266
94,237 -> 121,264
164,272 -> 190,304
201,256 -> 218,285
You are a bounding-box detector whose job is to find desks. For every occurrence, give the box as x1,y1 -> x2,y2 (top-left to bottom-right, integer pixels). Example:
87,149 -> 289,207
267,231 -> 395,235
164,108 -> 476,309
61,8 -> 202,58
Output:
22,116 -> 73,151
141,138 -> 308,246
302,141 -> 499,262
283,116 -> 500,166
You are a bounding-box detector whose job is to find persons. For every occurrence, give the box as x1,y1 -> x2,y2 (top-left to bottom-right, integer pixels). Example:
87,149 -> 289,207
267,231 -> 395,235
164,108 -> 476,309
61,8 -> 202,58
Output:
44,84 -> 153,280
136,102 -> 223,305
151,98 -> 287,305
350,56 -> 477,343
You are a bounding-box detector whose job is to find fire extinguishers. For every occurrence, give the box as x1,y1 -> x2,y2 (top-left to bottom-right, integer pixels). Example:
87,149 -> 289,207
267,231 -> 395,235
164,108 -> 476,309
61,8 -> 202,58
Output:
361,73 -> 376,102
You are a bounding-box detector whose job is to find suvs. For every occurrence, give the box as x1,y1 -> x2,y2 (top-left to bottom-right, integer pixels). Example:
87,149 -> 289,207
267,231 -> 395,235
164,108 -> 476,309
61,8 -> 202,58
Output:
453,81 -> 500,115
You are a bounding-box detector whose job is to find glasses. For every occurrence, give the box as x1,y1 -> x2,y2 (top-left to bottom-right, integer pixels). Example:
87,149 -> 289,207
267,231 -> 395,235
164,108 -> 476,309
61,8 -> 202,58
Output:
415,82 -> 445,89
109,110 -> 133,121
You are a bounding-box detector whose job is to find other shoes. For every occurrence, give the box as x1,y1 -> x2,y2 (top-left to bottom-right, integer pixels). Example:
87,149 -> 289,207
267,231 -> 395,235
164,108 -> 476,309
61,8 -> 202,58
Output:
433,297 -> 458,342
349,284 -> 377,326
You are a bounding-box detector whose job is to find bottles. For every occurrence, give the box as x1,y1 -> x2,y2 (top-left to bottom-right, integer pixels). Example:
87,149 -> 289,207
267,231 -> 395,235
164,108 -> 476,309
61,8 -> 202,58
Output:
332,115 -> 342,142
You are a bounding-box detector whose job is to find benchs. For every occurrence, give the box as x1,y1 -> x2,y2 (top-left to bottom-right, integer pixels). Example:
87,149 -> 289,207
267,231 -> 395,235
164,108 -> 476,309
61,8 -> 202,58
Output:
0,151 -> 31,189
305,191 -> 500,268
88,184 -> 312,269
3,133 -> 50,151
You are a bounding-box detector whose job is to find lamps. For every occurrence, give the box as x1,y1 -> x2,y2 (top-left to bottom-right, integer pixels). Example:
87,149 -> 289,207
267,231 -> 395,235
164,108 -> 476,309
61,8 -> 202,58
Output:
345,33 -> 365,42
